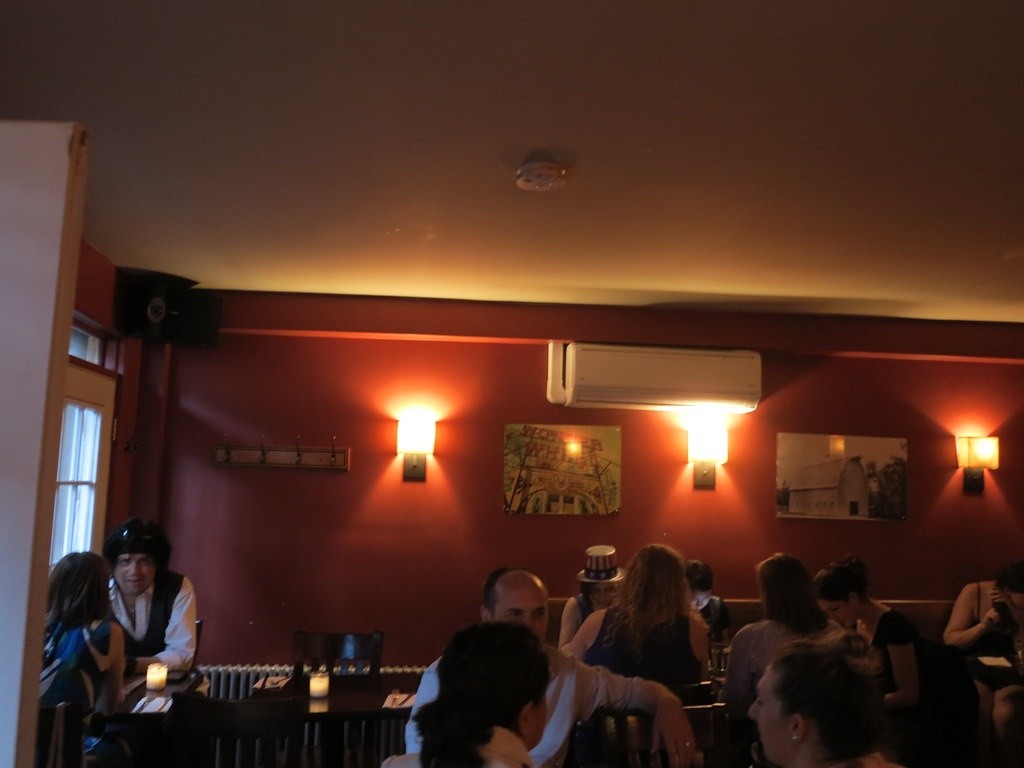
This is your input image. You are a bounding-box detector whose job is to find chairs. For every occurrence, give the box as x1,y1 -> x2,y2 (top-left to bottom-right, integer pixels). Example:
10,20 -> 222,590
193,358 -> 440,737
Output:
35,620 -> 980,768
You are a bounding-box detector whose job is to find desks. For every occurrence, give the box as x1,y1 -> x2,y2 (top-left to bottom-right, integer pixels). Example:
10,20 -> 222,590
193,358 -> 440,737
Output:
83,674 -> 420,768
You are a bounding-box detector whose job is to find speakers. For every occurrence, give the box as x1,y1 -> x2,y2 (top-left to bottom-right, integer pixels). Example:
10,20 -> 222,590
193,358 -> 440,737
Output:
114,275 -> 222,349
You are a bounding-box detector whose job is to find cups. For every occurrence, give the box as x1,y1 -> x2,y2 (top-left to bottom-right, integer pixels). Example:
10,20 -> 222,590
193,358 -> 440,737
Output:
309,670 -> 330,698
146,663 -> 168,690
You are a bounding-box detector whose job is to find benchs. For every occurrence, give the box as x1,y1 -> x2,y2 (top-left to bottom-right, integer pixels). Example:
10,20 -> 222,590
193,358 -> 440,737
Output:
544,597 -> 957,643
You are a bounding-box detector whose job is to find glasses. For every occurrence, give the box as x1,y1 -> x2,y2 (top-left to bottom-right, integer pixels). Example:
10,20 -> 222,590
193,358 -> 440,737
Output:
591,587 -> 619,596
118,530 -> 159,544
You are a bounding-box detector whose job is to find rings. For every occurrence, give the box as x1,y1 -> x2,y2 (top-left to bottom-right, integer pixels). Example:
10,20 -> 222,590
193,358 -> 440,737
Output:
685,741 -> 692,747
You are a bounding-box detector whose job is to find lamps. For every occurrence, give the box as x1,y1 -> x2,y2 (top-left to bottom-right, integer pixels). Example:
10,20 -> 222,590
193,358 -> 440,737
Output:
955,435 -> 1000,494
394,417 -> 435,482
681,420 -> 730,488
516,149 -> 569,192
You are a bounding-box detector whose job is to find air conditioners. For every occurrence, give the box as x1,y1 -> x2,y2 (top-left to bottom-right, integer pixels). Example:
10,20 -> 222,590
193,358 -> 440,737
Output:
564,342 -> 762,414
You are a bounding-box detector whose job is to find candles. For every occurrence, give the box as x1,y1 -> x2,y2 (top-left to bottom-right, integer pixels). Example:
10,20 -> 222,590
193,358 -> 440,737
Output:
310,671 -> 329,698
146,663 -> 167,690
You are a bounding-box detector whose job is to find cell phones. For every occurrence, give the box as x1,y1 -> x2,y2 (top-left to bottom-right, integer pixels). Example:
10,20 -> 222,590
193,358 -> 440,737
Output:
165,670 -> 188,684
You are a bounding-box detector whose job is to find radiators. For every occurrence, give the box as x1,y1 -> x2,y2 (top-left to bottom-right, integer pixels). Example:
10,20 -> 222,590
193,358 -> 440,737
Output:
195,661 -> 428,768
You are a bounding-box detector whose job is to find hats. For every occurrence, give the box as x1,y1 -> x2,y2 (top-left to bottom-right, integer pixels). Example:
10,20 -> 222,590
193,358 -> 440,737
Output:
577,543 -> 626,584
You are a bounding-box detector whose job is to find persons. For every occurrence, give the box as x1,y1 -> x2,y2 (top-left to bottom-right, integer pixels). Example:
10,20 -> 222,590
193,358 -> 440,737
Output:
380,544 -> 1024,767
37,552 -> 132,768
102,518 -> 198,681
